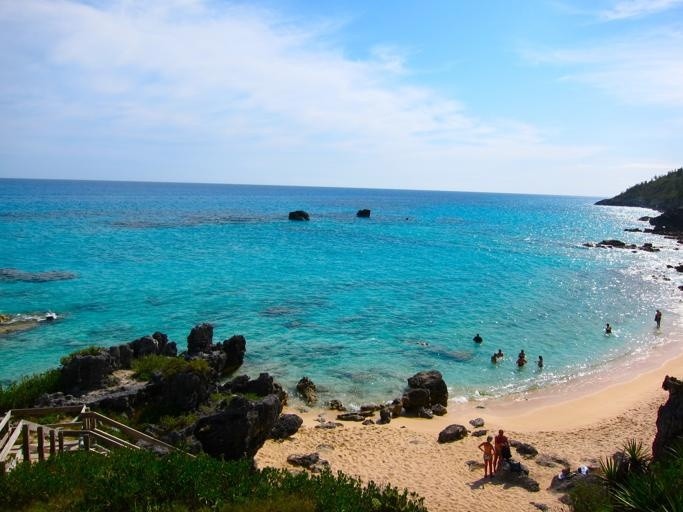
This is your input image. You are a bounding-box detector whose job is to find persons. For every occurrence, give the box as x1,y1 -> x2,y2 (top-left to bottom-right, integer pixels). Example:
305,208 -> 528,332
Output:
493,429 -> 508,473
497,349 -> 503,358
473,334 -> 482,343
491,353 -> 497,363
606,324 -> 612,333
654,310 -> 662,327
516,349 -> 543,367
478,435 -> 495,478
